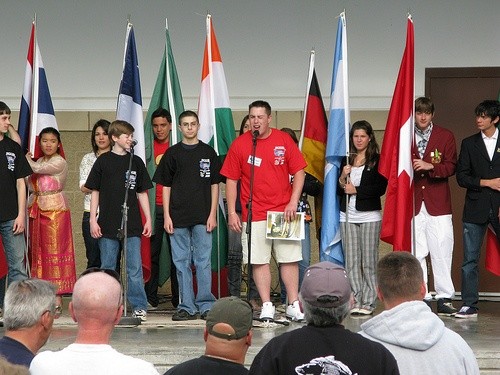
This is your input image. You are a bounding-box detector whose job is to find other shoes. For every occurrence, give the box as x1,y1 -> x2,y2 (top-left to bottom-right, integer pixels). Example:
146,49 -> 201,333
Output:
131,308 -> 148,321
147,302 -> 158,311
287,300 -> 304,322
200,310 -> 209,319
349,307 -> 374,315
259,302 -> 276,321
171,309 -> 198,321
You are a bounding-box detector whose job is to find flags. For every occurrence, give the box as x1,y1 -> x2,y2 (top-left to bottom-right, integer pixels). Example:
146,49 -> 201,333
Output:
19,12 -> 65,175
378,10 -> 418,258
109,9 -> 236,284
296,12 -> 351,266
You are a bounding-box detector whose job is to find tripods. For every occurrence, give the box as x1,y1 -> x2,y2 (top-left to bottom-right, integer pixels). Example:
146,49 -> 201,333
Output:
243,140 -> 290,325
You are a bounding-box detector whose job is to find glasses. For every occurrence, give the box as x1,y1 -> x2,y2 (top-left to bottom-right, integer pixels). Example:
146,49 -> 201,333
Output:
180,122 -> 198,126
53,305 -> 62,319
78,267 -> 121,286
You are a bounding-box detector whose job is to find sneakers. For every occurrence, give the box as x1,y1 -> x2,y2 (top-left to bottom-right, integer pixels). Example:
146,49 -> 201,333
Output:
455,305 -> 478,318
436,298 -> 459,316
423,299 -> 439,315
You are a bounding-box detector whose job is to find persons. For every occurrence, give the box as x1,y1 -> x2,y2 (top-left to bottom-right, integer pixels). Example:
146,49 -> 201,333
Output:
79,118 -> 122,277
225,114 -> 261,312
220,100 -> 308,322
0,251 -> 479,375
5,124 -> 22,146
144,108 -> 181,310
339,118 -> 388,315
84,120 -> 152,321
277,127 -> 312,309
24,127 -> 76,318
151,110 -> 218,321
0,100 -> 34,325
411,97 -> 460,317
455,100 -> 500,318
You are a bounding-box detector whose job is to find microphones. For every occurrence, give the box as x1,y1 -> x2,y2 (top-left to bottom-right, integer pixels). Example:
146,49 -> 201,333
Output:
130,139 -> 138,148
252,130 -> 259,140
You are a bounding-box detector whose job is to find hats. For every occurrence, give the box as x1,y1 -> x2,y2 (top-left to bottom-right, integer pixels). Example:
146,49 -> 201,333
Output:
207,295 -> 254,340
301,261 -> 351,308
